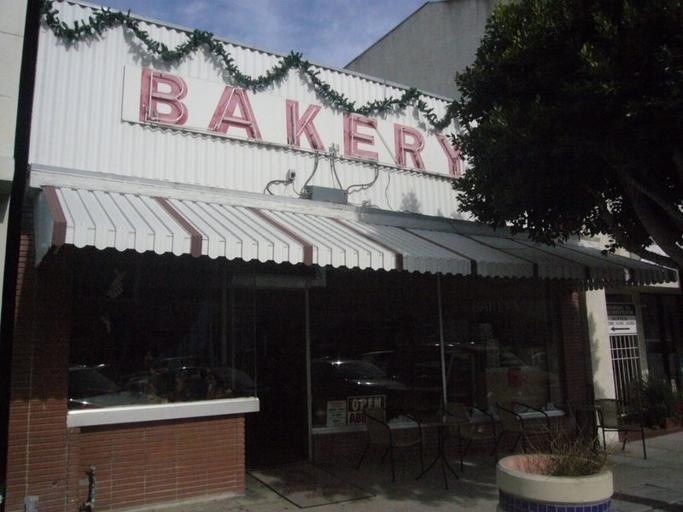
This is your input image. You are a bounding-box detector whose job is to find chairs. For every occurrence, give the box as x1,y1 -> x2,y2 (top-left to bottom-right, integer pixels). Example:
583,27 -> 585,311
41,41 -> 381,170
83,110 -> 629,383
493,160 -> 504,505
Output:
356,407 -> 424,483
490,401 -> 553,457
438,402 -> 499,471
593,399 -> 647,460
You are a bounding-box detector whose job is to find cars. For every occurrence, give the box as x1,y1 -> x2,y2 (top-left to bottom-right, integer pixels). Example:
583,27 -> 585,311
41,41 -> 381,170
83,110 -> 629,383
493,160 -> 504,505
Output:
68,342 -> 547,421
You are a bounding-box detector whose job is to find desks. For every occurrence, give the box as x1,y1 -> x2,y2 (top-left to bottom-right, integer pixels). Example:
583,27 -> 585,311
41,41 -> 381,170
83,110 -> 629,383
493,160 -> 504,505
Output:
555,402 -> 601,456
413,413 -> 469,490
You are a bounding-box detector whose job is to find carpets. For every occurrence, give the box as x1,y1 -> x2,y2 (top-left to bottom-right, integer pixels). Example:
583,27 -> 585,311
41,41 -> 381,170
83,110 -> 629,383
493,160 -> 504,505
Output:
248,462 -> 376,509
